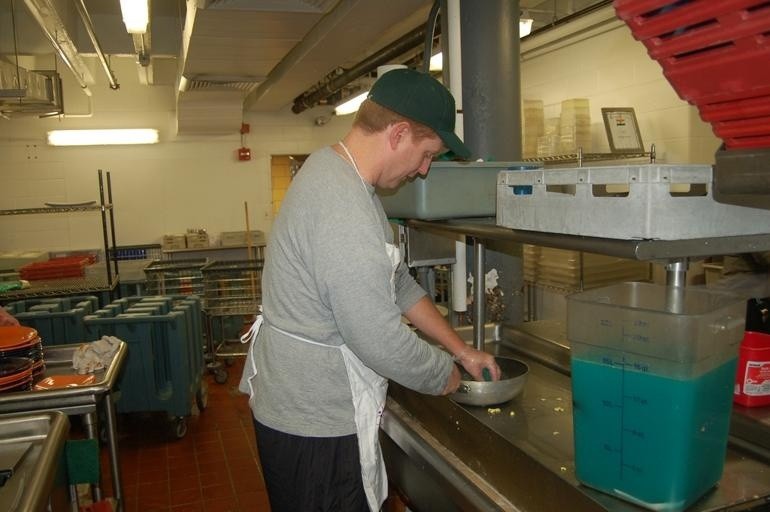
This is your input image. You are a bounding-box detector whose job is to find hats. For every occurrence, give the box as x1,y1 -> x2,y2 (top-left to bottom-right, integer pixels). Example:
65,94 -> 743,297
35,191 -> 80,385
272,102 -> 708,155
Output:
367,68 -> 473,157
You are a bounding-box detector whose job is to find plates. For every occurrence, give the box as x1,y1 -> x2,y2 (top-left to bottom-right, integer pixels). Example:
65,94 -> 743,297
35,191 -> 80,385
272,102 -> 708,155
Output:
0,358 -> 34,392
34,375 -> 96,390
0,325 -> 47,379
400,302 -> 448,324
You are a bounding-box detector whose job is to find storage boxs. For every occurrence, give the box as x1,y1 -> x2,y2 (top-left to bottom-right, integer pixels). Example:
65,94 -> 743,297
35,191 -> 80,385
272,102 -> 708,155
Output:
379,161 -> 542,220
222,230 -> 264,247
186,234 -> 209,248
163,236 -> 186,248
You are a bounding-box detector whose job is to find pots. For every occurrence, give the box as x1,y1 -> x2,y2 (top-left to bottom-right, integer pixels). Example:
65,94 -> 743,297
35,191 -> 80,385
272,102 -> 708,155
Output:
444,358 -> 527,405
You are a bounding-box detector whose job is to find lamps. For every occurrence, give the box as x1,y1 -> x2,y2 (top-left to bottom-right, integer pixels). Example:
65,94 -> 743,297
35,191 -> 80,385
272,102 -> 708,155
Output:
429,19 -> 534,71
120,0 -> 149,34
335,87 -> 369,116
46,128 -> 160,146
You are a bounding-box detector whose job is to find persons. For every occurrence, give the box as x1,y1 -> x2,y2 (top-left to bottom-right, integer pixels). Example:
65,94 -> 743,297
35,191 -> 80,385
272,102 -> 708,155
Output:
238,68 -> 504,512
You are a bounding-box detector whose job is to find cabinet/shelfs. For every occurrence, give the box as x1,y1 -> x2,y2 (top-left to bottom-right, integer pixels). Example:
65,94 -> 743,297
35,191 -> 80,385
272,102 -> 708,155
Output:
162,243 -> 267,274
0,169 -> 122,303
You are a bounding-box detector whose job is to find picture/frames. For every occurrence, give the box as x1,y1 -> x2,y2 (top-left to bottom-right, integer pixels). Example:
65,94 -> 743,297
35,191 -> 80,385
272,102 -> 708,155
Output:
602,107 -> 645,153
